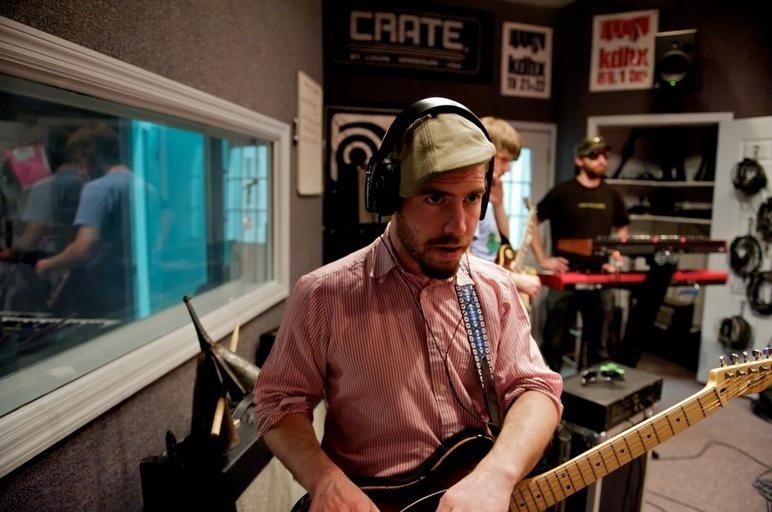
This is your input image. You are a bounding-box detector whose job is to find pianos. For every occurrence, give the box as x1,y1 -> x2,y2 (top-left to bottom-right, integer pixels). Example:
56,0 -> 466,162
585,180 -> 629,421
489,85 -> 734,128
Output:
538,236 -> 728,290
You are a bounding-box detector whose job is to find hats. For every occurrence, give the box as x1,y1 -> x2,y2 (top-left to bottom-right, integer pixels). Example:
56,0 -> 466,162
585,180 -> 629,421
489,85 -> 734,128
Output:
572,135 -> 611,156
398,112 -> 495,197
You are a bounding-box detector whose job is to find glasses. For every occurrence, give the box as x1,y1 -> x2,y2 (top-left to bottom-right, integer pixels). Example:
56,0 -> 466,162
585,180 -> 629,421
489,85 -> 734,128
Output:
585,150 -> 609,160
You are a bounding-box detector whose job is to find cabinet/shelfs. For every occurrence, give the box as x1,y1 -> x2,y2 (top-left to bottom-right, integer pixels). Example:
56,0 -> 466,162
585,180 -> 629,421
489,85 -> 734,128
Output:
595,177 -> 713,225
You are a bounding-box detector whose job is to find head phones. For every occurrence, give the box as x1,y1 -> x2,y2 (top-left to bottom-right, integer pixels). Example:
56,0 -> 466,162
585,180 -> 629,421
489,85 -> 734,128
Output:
364,96 -> 497,222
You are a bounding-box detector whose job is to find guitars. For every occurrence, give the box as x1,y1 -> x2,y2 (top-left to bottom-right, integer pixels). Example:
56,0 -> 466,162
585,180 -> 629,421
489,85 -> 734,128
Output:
290,345 -> 771,511
494,198 -> 538,315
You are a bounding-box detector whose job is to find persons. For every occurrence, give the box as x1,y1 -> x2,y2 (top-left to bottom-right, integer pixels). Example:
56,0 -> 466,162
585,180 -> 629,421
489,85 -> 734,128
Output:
0,122 -> 164,319
529,136 -> 630,373
462,119 -> 542,303
254,92 -> 566,512
